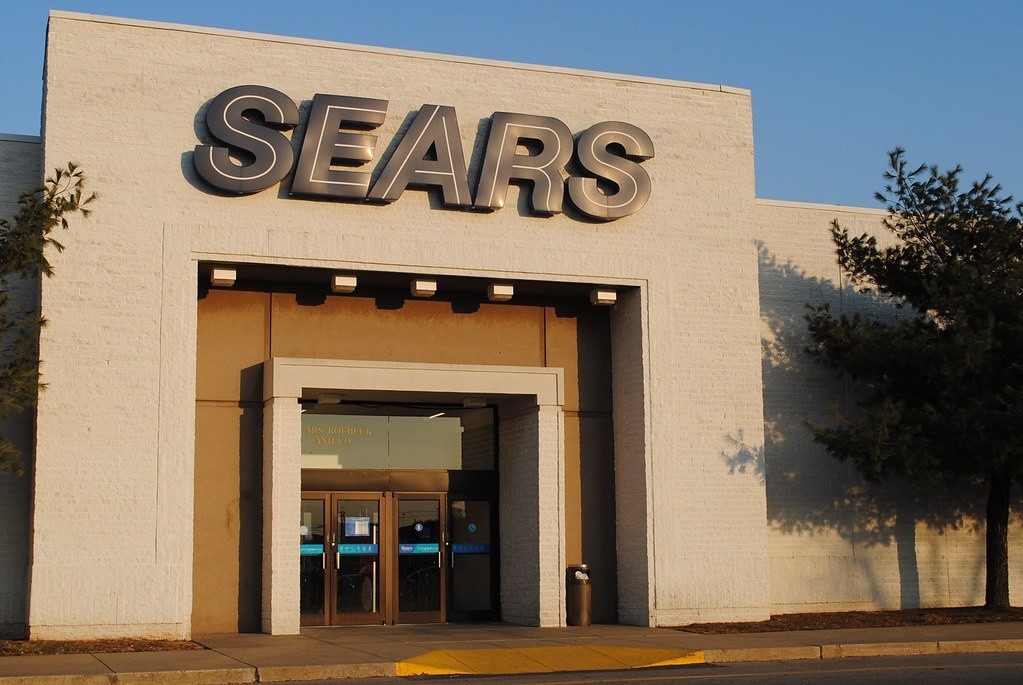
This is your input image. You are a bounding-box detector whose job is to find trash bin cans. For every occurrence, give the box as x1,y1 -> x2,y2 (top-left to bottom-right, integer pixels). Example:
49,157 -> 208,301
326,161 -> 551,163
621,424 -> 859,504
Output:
568,563 -> 591,626
